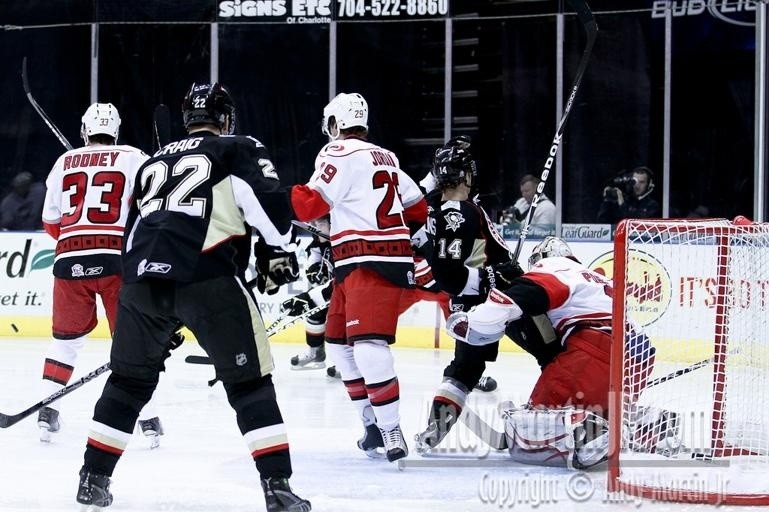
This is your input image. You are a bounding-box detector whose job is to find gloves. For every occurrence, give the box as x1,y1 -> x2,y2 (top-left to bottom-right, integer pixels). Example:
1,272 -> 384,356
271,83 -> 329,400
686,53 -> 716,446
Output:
480,262 -> 523,295
157,331 -> 186,373
253,242 -> 301,295
280,288 -> 321,319
444,286 -> 522,347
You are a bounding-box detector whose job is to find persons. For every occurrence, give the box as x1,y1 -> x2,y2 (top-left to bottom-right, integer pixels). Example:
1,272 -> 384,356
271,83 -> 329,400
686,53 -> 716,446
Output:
445,237 -> 714,471
37,102 -> 164,450
256,91 -> 429,462
397,249 -> 498,393
280,215 -> 343,377
1,172 -> 45,228
77,82 -> 312,512
416,137 -> 564,454
597,166 -> 662,222
499,176 -> 554,224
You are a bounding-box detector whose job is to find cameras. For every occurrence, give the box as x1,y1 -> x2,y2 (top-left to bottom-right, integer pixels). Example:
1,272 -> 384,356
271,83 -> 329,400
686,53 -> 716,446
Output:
501,206 -> 522,228
606,169 -> 635,202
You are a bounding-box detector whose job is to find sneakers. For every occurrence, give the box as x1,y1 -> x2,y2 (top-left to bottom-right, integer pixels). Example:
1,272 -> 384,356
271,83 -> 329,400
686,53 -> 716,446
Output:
377,420 -> 408,463
474,376 -> 497,392
326,364 -> 341,378
36,407 -> 61,432
420,421 -> 449,453
138,415 -> 164,437
575,423 -> 623,466
629,411 -> 681,454
291,346 -> 327,367
77,466 -> 114,508
257,476 -> 312,512
356,425 -> 387,451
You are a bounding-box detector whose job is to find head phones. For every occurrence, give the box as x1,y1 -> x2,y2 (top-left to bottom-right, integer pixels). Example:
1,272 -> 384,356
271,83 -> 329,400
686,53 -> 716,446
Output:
638,166 -> 655,200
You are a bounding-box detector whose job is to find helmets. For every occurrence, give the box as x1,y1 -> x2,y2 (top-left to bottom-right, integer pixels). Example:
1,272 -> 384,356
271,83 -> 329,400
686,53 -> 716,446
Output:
320,92 -> 370,138
181,81 -> 237,134
431,135 -> 472,191
13,172 -> 34,192
527,235 -> 573,271
79,102 -> 122,146
